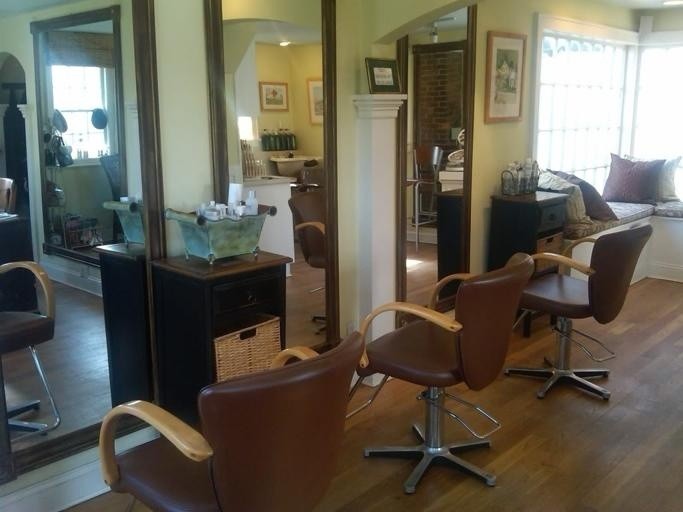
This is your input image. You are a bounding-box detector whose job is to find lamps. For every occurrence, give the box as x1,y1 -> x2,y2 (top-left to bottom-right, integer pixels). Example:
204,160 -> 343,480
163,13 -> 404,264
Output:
235,116 -> 254,183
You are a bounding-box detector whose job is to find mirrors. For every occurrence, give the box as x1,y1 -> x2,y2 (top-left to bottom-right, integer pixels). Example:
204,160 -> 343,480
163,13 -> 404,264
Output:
203,1 -> 340,356
29,5 -> 127,268
0,0 -> 167,485
394,4 -> 476,330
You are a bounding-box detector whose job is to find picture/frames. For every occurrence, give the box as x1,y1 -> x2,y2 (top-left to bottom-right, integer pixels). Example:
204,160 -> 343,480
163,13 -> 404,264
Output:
259,81 -> 288,112
483,30 -> 526,124
365,57 -> 403,93
308,80 -> 323,123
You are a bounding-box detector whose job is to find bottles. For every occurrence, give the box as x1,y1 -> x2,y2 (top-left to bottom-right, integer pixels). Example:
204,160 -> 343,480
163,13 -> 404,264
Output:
267,129 -> 275,150
272,130 -> 281,151
278,128 -> 286,150
284,128 -> 292,150
261,129 -> 270,150
289,129 -> 297,150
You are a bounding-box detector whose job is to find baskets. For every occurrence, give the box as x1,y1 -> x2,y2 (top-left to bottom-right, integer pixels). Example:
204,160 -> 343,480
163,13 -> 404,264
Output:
212,312 -> 281,384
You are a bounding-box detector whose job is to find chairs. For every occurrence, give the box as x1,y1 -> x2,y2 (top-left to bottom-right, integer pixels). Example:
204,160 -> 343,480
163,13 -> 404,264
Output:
504,224 -> 653,401
407,146 -> 439,252
98,331 -> 365,512
288,191 -> 325,336
350,252 -> 534,495
0,261 -> 61,444
99,152 -> 126,242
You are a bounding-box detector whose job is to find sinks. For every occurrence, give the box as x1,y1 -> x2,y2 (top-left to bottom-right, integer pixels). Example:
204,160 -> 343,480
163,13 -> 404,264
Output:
242,175 -> 280,181
270,155 -> 308,176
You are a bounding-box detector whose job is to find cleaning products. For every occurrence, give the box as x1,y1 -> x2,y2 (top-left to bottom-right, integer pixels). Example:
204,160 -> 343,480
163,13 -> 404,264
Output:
262,129 -> 298,151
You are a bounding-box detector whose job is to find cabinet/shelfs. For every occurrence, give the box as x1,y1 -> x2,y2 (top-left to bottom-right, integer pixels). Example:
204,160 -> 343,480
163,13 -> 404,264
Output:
0,213 -> 38,312
93,242 -> 154,409
488,190 -> 576,338
431,188 -> 464,300
150,246 -> 293,438
243,175 -> 296,278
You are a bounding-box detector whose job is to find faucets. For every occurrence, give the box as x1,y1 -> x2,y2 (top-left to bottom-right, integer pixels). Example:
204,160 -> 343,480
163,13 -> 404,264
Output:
287,152 -> 295,157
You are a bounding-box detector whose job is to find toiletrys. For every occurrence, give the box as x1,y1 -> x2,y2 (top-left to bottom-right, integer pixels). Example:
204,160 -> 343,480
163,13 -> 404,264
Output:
279,128 -> 287,150
262,128 -> 269,151
284,129 -> 291,150
503,158 -> 539,179
246,191 -> 258,216
236,200 -> 245,217
269,129 -> 276,151
196,190 -> 258,221
290,128 -> 297,150
273,129 -> 282,151
204,201 -> 219,221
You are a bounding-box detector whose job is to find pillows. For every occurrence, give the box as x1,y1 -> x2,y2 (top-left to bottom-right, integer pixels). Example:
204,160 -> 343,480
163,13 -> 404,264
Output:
537,170 -> 593,224
601,153 -> 666,206
623,154 -> 680,201
546,168 -> 618,221
